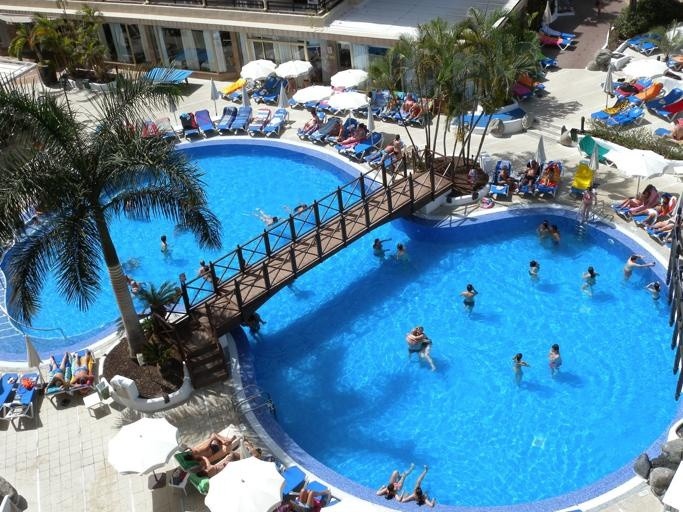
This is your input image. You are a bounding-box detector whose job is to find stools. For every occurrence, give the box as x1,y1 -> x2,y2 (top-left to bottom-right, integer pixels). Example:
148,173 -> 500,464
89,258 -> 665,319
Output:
168,471 -> 190,496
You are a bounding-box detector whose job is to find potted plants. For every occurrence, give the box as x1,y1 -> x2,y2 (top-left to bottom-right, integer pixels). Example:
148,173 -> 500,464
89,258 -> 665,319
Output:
9,17 -> 60,85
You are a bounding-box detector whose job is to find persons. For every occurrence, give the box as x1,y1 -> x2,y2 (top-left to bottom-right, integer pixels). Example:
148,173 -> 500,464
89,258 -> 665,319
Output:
376,482 -> 436,508
184,432 -> 262,478
468,117 -> 679,242
276,488 -> 332,510
125,204 -> 308,333
45,350 -> 95,393
373,238 -> 661,367
300,93 -> 429,177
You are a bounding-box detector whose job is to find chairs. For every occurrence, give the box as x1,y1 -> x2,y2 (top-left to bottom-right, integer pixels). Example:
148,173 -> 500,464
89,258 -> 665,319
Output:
67,348 -> 97,399
648,88 -> 682,122
188,444 -> 272,496
5,374 -> 42,422
630,32 -> 682,71
174,424 -> 244,476
542,27 -> 577,39
539,35 -> 573,51
281,466 -> 308,500
297,482 -> 340,512
489,160 -> 513,196
576,134 -> 619,167
594,96 -> 644,131
519,160 -> 545,197
1,373 -> 17,420
45,352 -> 72,410
538,162 -> 564,200
541,56 -> 559,69
518,75 -> 544,96
572,162 -> 596,196
610,76 -> 666,106
514,83 -> 531,102
612,188 -> 681,245
144,77 -> 434,172
654,127 -> 673,138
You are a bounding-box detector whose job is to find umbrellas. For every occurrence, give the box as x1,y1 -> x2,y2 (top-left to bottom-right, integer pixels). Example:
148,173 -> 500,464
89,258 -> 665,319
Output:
25,334 -> 46,383
242,85 -> 251,108
278,83 -> 289,109
331,68 -> 369,88
623,59 -> 668,78
293,86 -> 333,109
603,66 -> 614,109
210,77 -> 219,116
240,59 -> 277,82
275,60 -> 313,85
